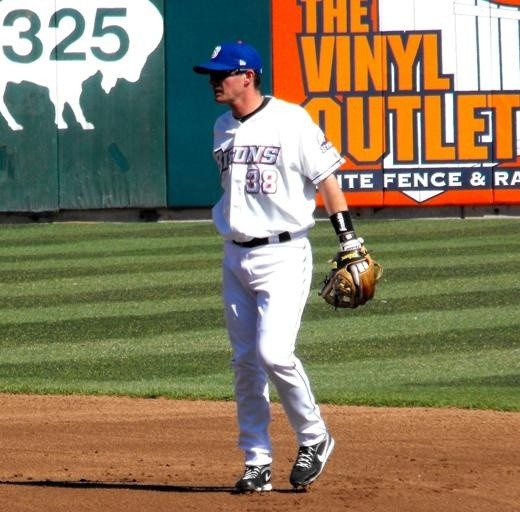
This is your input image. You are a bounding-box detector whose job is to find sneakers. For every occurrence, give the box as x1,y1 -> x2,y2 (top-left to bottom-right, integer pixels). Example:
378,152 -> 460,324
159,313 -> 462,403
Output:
235,464 -> 273,492
289,432 -> 335,488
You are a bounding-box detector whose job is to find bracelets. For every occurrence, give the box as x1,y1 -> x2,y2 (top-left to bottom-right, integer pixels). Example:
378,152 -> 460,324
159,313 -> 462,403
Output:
329,210 -> 354,234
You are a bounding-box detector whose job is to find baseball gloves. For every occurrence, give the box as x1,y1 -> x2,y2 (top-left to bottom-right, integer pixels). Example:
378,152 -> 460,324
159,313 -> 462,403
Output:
317,245 -> 383,309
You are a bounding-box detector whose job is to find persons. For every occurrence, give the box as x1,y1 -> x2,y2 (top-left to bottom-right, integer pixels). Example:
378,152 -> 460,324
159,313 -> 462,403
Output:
194,41 -> 369,492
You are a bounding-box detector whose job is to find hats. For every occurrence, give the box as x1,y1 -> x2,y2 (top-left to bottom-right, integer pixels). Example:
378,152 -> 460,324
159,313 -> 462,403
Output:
192,41 -> 264,75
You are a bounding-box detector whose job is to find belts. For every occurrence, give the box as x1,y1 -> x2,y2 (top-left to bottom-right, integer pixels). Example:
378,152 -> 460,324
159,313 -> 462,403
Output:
231,230 -> 292,248
